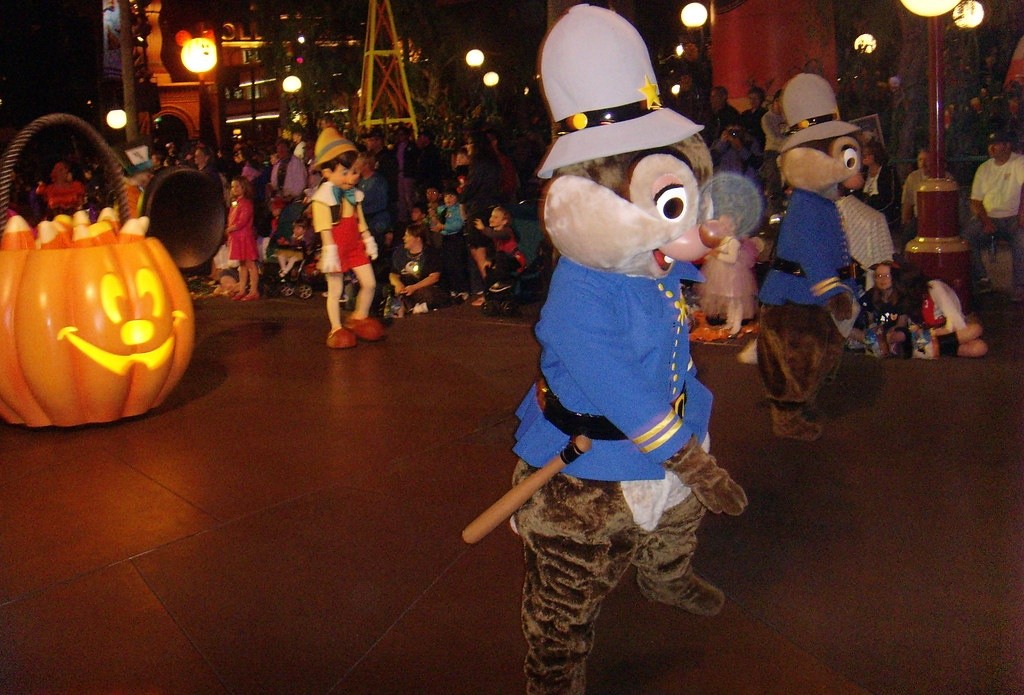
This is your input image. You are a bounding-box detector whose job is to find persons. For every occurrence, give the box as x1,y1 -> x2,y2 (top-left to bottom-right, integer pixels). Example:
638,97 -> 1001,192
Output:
655,42 -> 1023,362
8,116 -> 554,315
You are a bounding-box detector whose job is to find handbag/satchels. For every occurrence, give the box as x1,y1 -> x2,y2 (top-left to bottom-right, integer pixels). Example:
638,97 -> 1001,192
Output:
911,330 -> 940,360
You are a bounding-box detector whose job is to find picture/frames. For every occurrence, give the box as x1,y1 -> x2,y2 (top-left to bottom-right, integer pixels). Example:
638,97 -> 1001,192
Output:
849,114 -> 884,153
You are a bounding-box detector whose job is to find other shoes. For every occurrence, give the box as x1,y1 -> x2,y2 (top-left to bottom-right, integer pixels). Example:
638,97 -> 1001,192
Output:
232,290 -> 248,301
453,292 -> 470,302
974,277 -> 993,294
240,289 -> 260,303
1010,284 -> 1024,302
472,297 -> 487,306
890,342 -> 901,355
489,282 -> 514,292
414,302 -> 429,315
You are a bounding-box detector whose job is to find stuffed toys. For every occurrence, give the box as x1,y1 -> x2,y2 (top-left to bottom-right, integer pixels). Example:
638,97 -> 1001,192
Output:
310,127 -> 384,349
510,4 -> 747,695
754,74 -> 865,442
887,320 -> 987,360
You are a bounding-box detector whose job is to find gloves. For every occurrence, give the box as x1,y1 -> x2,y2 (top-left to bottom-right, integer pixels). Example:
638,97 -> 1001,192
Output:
917,328 -> 937,349
908,323 -> 923,338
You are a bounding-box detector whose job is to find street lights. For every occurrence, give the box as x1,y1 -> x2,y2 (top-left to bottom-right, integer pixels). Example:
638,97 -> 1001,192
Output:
465,49 -> 500,125
670,3 -> 709,111
181,36 -> 224,160
900,0 -> 975,321
103,100 -> 132,153
281,75 -> 303,129
852,33 -> 877,107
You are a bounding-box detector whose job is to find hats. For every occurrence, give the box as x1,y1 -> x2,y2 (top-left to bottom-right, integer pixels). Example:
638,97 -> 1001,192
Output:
987,133 -> 1010,143
361,126 -> 384,138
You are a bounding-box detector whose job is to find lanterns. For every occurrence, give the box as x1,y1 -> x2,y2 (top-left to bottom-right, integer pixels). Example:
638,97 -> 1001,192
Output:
0,208 -> 196,429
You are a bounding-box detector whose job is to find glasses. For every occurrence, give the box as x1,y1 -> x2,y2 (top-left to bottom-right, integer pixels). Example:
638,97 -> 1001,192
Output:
427,191 -> 436,195
874,274 -> 891,279
